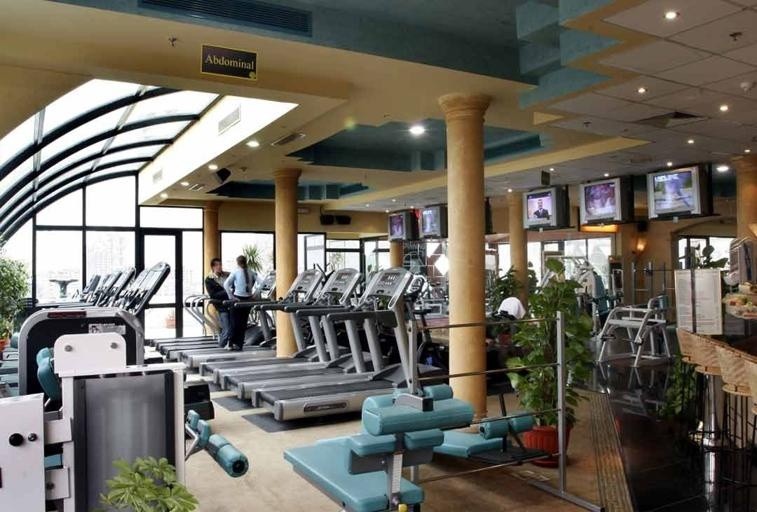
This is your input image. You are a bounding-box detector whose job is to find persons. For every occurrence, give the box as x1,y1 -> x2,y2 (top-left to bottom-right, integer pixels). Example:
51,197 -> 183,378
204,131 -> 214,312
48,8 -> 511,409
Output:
533,198 -> 550,221
392,215 -> 403,237
222,255 -> 264,352
205,257 -> 231,349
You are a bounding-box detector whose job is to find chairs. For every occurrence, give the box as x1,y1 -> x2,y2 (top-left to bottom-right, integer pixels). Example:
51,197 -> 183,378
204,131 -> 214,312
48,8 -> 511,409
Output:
676,327 -> 757,512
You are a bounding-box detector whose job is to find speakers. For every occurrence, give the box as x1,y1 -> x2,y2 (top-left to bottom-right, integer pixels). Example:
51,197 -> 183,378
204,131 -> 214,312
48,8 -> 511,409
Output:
214,168 -> 231,184
637,219 -> 648,233
320,215 -> 334,225
336,215 -> 351,224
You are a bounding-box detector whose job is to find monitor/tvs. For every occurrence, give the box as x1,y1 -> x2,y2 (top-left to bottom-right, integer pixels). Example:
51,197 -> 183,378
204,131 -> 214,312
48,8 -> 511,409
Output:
418,205 -> 447,239
646,165 -> 714,221
388,211 -> 417,241
579,178 -> 634,226
485,201 -> 493,234
522,187 -> 570,230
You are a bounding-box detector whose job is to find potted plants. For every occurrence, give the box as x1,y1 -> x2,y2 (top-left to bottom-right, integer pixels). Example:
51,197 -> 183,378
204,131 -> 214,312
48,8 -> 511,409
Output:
0,255 -> 33,353
504,258 -> 594,462
483,269 -> 524,345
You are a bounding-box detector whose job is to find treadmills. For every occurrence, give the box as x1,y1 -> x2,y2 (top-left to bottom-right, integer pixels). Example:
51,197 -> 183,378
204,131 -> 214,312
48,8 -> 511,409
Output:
0,262 -> 170,398
149,270 -> 443,422
